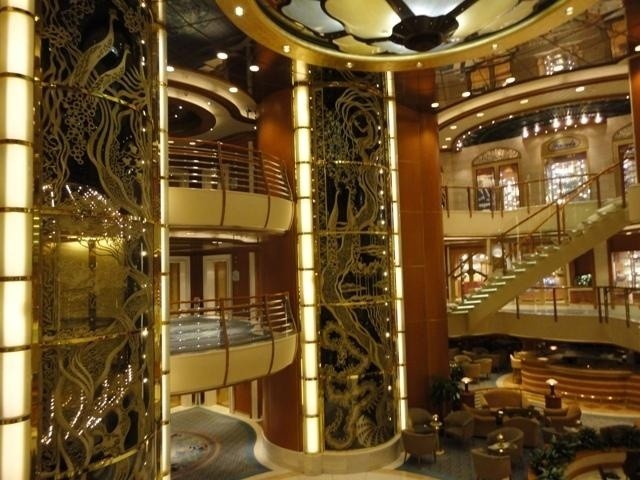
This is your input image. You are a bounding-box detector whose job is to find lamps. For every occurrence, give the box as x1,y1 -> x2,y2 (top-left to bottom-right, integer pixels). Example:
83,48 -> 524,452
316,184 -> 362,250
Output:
215,0 -> 599,71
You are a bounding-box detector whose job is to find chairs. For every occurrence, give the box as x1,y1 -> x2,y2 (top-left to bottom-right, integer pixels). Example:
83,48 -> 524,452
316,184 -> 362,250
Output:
398,402 -> 583,480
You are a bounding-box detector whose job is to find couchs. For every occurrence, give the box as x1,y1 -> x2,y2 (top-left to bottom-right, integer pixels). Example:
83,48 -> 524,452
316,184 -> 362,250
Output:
479,389 -> 535,416
